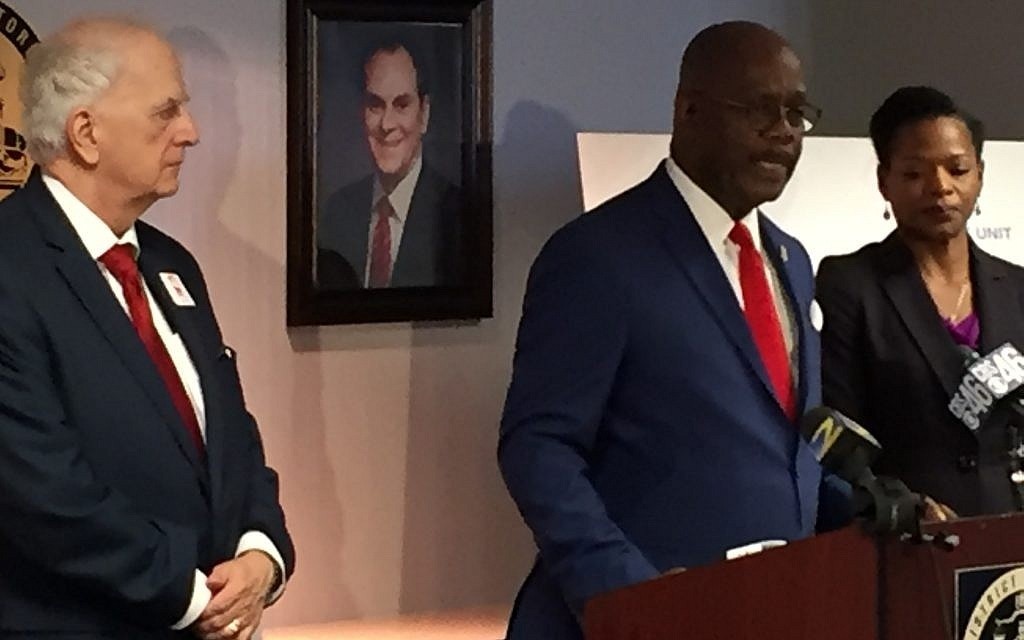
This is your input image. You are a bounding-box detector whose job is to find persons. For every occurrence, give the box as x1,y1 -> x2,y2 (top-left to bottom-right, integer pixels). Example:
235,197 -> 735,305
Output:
496,18 -> 954,640
0,17 -> 297,640
814,85 -> 1023,519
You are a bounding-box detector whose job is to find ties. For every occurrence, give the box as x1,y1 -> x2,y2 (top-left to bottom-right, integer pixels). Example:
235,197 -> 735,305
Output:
97,244 -> 207,467
368,197 -> 394,289
729,221 -> 796,419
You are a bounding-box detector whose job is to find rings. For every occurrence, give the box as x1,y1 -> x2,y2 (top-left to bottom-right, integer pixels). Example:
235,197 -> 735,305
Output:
229,619 -> 240,633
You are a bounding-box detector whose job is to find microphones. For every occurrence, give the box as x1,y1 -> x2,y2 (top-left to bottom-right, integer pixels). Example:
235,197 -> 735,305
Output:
938,342 -> 1024,436
799,406 -> 919,538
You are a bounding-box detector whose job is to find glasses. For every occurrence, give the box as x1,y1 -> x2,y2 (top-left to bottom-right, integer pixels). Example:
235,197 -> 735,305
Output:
706,94 -> 823,136
